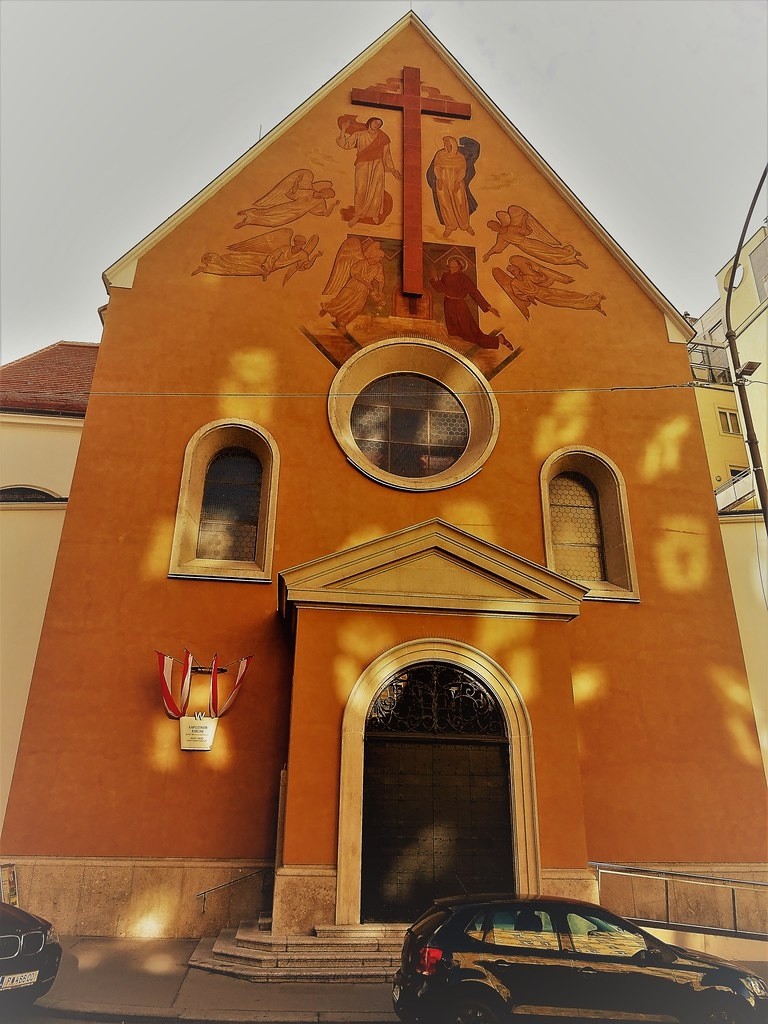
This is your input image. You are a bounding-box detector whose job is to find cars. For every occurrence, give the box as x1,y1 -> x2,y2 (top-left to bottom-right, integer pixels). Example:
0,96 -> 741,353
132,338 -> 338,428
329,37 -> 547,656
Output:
0,901 -> 62,1006
389,892 -> 768,1024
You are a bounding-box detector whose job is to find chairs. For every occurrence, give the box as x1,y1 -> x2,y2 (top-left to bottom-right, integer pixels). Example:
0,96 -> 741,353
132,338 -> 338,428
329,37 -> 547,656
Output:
514,912 -> 543,933
